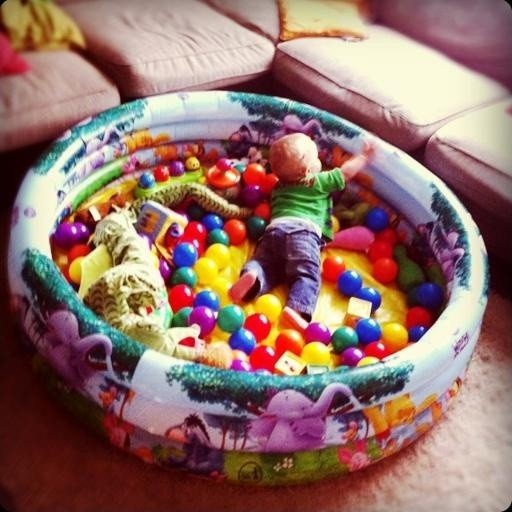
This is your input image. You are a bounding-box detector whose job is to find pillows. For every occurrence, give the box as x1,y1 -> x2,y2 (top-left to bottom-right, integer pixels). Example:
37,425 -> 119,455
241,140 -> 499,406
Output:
0,32 -> 31,77
278,1 -> 366,40
0,0 -> 85,52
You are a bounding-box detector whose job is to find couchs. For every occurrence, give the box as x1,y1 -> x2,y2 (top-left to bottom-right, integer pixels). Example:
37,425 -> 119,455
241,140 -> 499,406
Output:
0,0 -> 512,264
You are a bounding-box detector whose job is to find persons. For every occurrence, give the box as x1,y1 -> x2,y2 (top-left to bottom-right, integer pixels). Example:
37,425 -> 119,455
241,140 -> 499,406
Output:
227,134 -> 374,329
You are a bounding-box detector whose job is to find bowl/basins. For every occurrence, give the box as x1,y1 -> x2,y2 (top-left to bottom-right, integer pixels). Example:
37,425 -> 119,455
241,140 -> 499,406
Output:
9,90 -> 487,485
